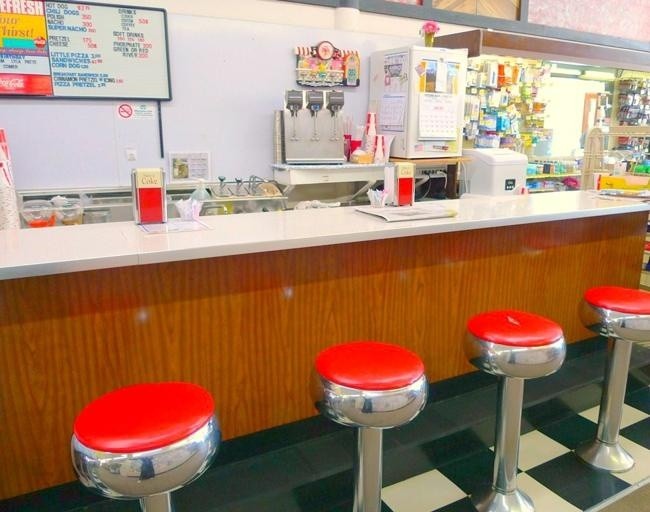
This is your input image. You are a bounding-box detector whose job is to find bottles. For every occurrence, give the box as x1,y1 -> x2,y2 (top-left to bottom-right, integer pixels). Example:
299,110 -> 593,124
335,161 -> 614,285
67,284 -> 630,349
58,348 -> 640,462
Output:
346,53 -> 358,85
534,159 -> 567,174
344,134 -> 351,161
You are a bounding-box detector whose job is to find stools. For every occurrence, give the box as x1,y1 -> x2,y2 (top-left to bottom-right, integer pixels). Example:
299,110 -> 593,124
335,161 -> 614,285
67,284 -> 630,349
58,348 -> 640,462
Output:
462,310 -> 567,512
309,341 -> 428,512
575,286 -> 650,474
69,381 -> 221,512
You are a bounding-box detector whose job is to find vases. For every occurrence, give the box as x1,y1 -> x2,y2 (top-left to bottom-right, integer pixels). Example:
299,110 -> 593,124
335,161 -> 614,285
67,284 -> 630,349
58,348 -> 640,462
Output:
425,32 -> 435,47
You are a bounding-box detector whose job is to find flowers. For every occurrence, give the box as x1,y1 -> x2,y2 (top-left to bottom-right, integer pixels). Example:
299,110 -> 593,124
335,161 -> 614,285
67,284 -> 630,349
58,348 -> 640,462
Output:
419,21 -> 440,36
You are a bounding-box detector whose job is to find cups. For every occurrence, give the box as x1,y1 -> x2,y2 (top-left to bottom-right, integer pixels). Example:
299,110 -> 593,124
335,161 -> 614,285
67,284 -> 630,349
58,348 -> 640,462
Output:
351,140 -> 362,153
21,197 -> 53,227
0,128 -> 21,230
54,197 -> 83,225
364,111 -> 386,163
